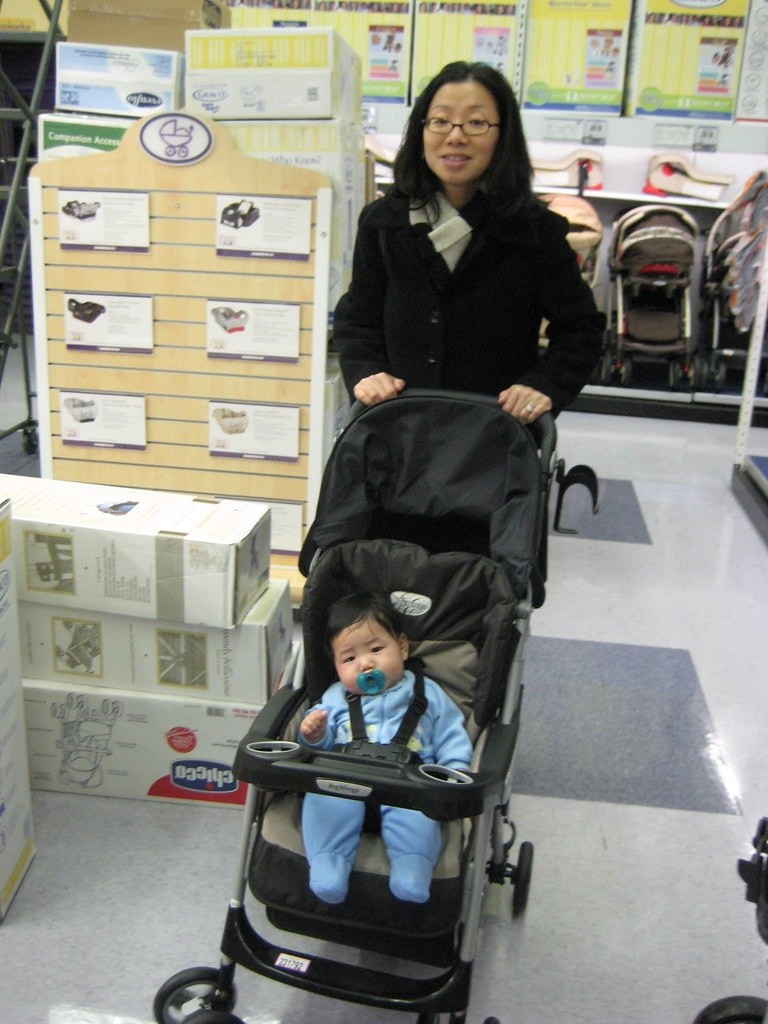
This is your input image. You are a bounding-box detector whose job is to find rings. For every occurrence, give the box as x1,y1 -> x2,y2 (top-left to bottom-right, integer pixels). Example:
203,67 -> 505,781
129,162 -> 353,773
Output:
526,404 -> 533,412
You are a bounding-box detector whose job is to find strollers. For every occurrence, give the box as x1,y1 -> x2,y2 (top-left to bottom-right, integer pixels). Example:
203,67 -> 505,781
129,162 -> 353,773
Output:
553,174 -> 767,397
149,388 -> 558,1024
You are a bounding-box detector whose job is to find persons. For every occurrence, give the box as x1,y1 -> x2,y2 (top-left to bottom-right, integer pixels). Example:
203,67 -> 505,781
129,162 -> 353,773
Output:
333,60 -> 609,450
296,591 -> 473,905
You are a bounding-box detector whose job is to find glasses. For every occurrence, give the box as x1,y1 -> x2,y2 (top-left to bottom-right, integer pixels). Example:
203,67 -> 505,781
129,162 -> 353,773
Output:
420,117 -> 500,136
